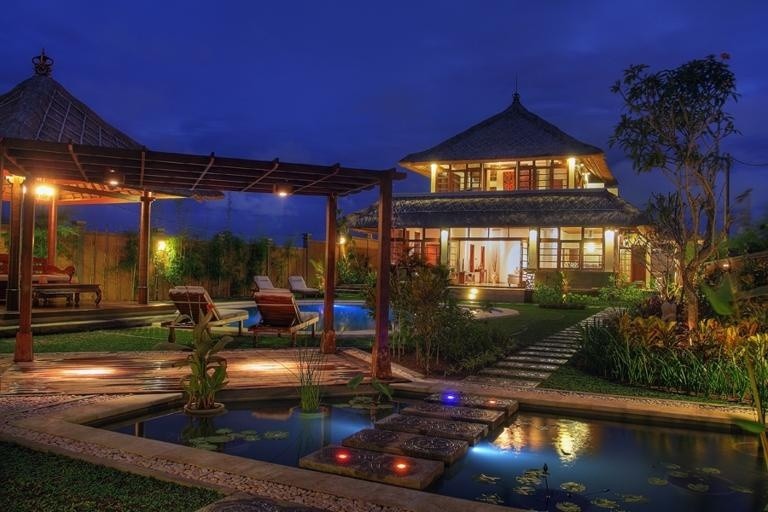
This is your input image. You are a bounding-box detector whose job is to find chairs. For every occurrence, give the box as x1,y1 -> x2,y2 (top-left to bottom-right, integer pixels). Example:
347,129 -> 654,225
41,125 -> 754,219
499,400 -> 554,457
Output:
161,286 -> 248,343
287,275 -> 320,299
251,275 -> 294,298
250,290 -> 320,346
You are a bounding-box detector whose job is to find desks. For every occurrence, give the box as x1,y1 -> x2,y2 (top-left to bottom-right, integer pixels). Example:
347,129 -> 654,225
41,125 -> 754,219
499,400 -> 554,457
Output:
31,282 -> 102,306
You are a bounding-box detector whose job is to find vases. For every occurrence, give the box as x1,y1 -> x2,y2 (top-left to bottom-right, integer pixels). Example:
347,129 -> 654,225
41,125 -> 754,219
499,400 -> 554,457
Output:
184,402 -> 224,416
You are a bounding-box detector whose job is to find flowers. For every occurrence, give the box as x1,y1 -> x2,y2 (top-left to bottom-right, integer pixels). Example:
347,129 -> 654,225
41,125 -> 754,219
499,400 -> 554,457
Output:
175,302 -> 233,409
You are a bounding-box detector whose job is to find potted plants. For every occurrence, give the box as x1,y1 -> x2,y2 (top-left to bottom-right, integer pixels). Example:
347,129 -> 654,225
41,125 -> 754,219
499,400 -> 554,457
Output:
266,348 -> 329,419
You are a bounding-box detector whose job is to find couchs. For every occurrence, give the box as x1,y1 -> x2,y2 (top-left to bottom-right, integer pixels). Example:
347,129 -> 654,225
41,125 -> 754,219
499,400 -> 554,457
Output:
507,274 -> 525,287
0,254 -> 75,306
